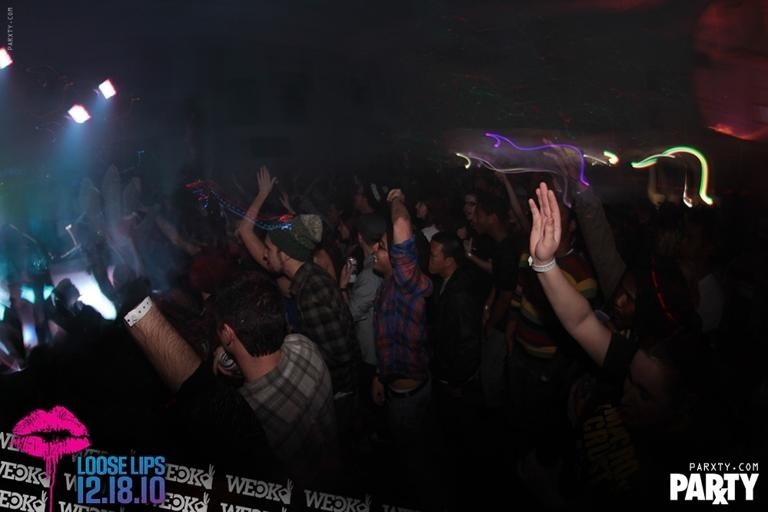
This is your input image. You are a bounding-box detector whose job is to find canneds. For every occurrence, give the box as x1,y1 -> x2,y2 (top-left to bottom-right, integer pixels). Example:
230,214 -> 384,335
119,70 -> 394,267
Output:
346,255 -> 359,283
220,352 -> 242,376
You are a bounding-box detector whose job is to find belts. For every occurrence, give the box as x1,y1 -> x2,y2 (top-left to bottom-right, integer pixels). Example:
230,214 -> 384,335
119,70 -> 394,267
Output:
387,373 -> 429,398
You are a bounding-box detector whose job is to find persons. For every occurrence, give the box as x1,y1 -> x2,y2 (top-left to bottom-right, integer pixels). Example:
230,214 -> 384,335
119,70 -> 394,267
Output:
0,163 -> 767,512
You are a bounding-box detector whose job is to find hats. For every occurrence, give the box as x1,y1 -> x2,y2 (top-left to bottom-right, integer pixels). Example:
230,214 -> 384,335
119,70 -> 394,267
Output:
364,183 -> 386,209
267,213 -> 323,261
